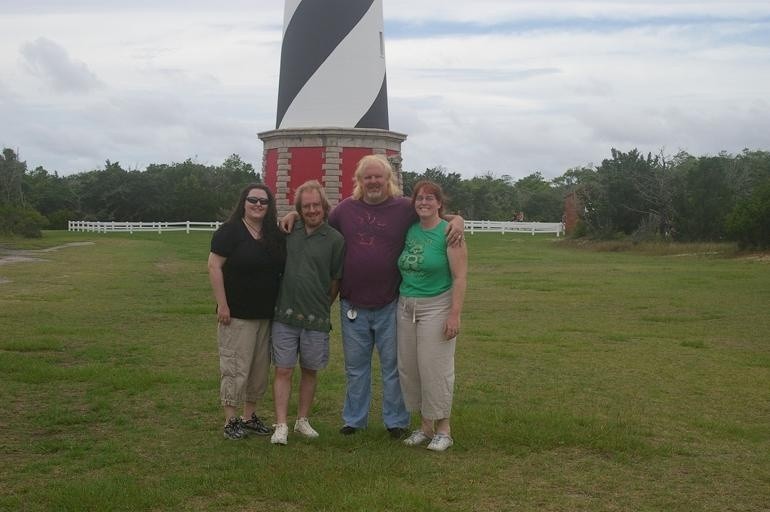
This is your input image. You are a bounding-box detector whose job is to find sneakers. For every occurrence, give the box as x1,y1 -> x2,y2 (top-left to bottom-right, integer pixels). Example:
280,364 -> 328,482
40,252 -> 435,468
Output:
389,428 -> 405,441
338,426 -> 355,435
270,423 -> 289,445
426,433 -> 454,452
403,429 -> 433,447
293,416 -> 319,439
223,416 -> 249,441
239,412 -> 274,436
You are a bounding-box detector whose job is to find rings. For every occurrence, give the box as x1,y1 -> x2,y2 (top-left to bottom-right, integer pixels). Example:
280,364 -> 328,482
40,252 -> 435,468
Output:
457,235 -> 462,240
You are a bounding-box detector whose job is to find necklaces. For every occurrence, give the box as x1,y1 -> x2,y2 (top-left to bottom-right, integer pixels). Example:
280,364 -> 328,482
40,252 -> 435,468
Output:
242,216 -> 264,237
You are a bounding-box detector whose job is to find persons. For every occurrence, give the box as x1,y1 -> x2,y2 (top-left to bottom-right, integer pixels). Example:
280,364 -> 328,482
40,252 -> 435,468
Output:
270,176 -> 347,445
396,179 -> 468,454
209,182 -> 288,440
280,153 -> 465,440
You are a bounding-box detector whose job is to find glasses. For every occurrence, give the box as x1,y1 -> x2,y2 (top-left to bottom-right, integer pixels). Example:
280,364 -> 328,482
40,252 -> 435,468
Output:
244,196 -> 269,206
